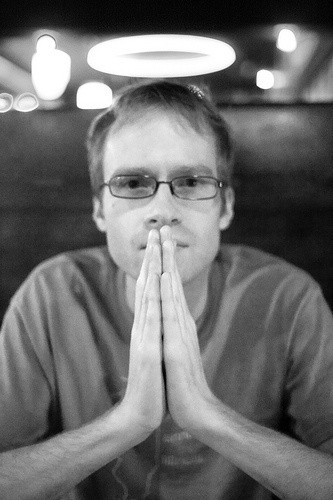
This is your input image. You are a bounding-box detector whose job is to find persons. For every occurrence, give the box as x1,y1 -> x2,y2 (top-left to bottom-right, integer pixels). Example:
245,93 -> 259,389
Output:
1,77 -> 332,500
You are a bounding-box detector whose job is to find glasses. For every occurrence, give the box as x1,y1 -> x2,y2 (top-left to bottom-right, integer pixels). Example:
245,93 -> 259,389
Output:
99,172 -> 229,201
